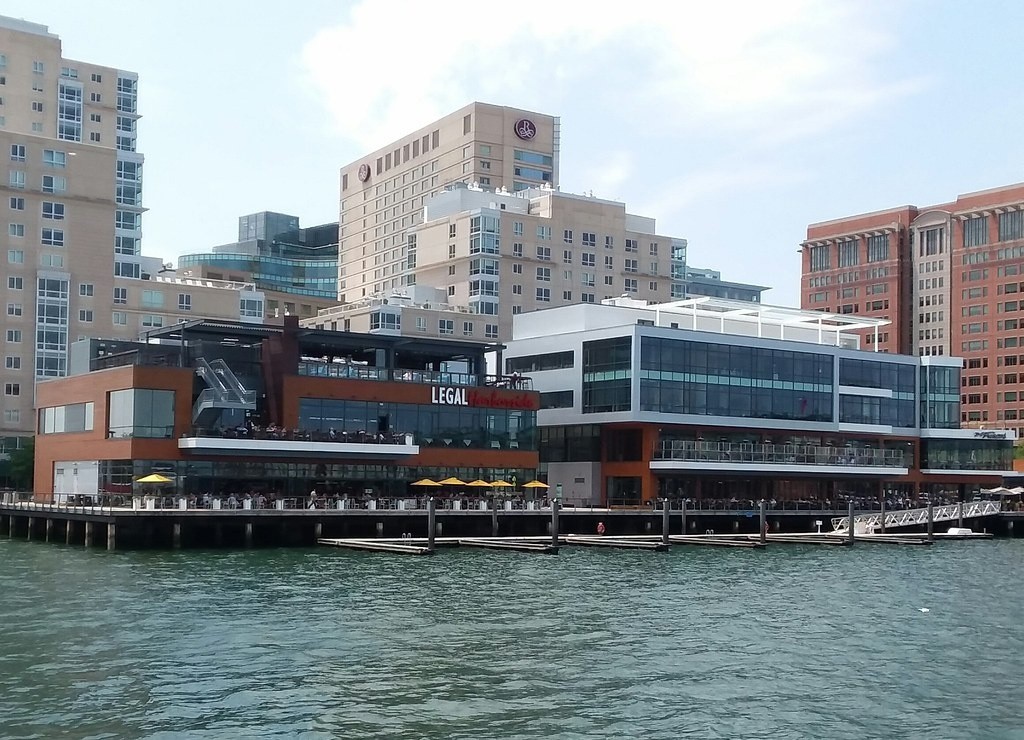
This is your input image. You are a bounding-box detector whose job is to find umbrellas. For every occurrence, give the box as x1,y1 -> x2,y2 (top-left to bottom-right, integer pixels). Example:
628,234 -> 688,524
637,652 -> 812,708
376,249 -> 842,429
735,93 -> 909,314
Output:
437,476 -> 466,499
410,478 -> 442,496
490,479 -> 514,500
981,486 -> 1024,501
136,473 -> 173,499
521,480 -> 551,500
466,479 -> 493,500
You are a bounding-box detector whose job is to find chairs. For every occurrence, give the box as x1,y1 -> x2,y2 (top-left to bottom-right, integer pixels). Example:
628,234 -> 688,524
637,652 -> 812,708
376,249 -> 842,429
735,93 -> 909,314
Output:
298,364 -> 476,384
66,492 -> 548,509
223,426 -> 406,445
652,495 -> 1024,510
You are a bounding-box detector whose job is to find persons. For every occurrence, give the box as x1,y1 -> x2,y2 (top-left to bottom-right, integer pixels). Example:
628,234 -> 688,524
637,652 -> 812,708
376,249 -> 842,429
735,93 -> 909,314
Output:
449,491 -> 459,502
236,416 -> 287,441
330,427 -> 336,439
361,492 -> 371,508
509,372 -> 518,390
308,488 -> 317,509
244,490 -> 264,509
189,492 -> 196,506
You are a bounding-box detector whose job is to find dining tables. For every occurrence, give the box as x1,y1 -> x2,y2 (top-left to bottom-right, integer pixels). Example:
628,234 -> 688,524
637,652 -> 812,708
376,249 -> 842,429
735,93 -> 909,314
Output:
420,437 -> 518,449
